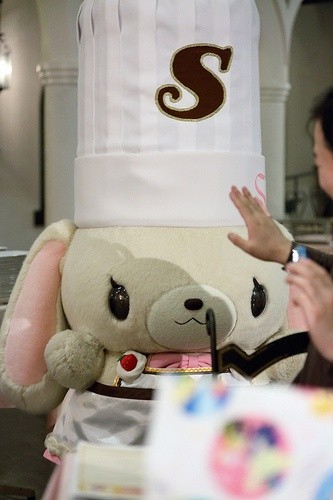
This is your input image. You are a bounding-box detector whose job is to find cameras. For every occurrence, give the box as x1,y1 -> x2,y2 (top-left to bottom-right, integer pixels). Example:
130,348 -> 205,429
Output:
292,247 -> 307,263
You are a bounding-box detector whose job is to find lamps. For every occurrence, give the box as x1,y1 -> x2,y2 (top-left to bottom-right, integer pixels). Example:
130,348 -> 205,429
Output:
0,32 -> 13,91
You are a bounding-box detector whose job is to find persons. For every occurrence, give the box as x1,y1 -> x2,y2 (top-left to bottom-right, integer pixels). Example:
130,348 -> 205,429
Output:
227,83 -> 332,390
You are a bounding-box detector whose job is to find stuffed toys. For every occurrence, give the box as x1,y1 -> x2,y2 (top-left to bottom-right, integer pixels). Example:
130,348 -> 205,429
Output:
0,0 -> 310,500
